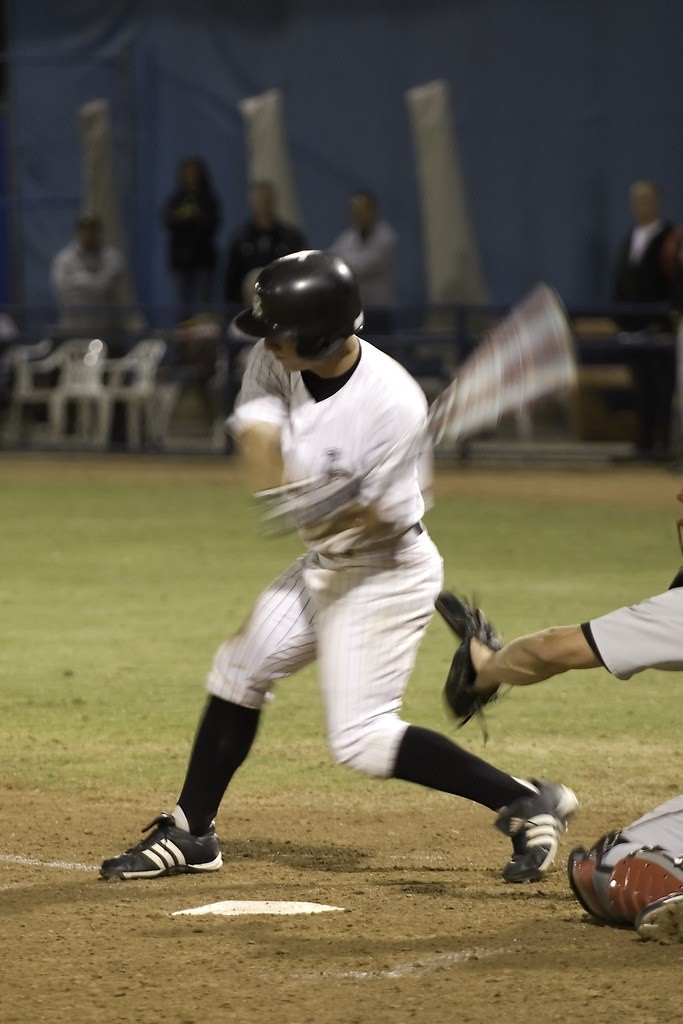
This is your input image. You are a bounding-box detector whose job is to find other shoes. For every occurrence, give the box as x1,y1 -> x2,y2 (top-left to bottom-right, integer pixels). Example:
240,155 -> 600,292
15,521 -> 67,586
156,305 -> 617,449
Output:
638,893 -> 683,945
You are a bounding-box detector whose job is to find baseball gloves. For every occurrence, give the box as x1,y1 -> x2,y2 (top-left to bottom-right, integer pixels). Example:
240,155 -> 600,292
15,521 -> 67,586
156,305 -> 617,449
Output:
433,589 -> 502,725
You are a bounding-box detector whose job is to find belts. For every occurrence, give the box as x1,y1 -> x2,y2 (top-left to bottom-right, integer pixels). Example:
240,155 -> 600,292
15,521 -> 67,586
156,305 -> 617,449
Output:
342,523 -> 422,556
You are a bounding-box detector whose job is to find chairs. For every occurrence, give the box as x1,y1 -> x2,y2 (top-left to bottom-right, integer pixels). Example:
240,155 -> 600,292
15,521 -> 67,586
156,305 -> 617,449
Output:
0,336 -> 170,453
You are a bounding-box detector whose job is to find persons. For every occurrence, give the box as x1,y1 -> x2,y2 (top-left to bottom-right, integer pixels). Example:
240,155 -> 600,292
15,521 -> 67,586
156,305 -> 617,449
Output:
604,177 -> 683,471
432,571 -> 683,946
96,250 -> 577,883
52,153 -> 400,452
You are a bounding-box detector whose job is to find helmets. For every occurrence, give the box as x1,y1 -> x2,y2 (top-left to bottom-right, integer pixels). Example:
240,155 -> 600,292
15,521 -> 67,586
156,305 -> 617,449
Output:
234,249 -> 365,359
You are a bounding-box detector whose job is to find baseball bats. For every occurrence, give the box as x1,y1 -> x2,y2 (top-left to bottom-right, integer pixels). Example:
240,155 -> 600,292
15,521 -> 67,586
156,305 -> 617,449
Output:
252,281 -> 582,542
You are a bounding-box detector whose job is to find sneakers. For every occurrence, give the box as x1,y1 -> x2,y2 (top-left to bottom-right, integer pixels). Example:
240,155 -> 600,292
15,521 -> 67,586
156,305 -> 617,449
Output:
496,778 -> 579,881
99,812 -> 224,880
